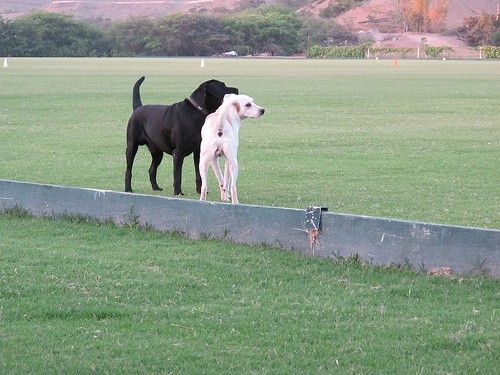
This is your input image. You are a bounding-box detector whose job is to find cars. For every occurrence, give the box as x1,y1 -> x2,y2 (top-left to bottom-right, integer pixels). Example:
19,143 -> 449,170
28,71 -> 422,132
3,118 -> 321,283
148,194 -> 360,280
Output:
212,49 -> 239,56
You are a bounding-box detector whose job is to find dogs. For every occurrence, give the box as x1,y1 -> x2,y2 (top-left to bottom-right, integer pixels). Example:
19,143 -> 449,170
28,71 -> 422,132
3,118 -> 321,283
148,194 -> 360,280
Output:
123,76 -> 239,196
200,94 -> 264,205
